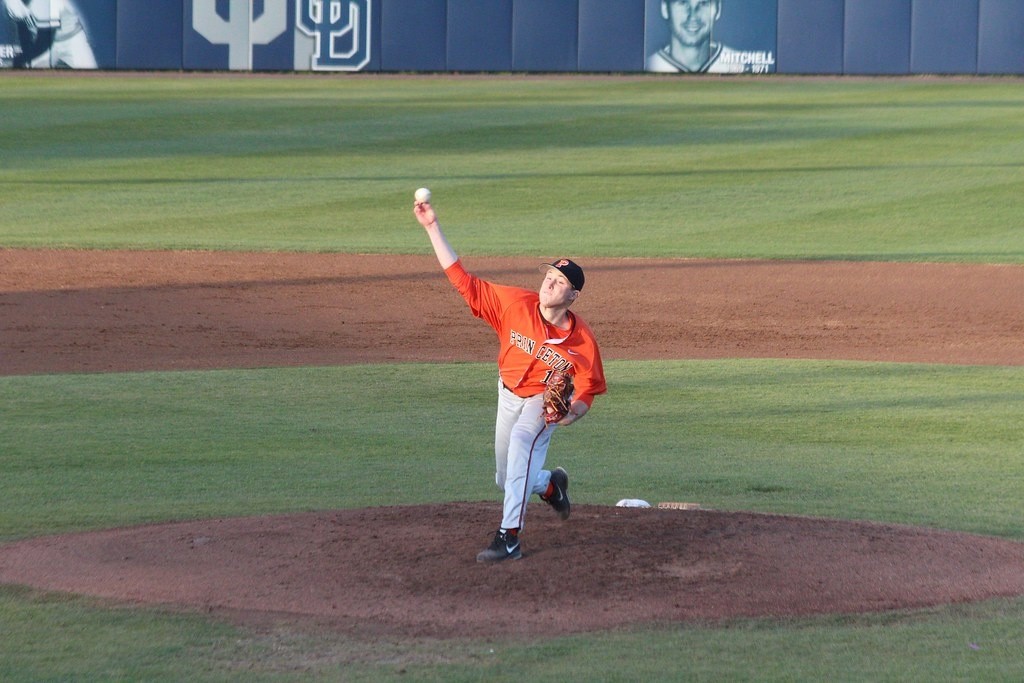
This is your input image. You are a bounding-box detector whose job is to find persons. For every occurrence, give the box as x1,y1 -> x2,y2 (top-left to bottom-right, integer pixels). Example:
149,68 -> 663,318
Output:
643,0 -> 751,75
5,0 -> 99,71
413,199 -> 608,563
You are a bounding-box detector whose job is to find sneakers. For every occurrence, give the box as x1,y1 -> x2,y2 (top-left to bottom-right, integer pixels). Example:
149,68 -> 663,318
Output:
476,528 -> 523,564
539,467 -> 571,521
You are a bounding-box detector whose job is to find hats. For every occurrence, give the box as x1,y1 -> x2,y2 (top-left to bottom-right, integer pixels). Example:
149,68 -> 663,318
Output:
537,258 -> 585,292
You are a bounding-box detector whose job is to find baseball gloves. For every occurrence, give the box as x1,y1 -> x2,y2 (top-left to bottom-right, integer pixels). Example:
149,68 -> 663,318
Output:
541,373 -> 573,426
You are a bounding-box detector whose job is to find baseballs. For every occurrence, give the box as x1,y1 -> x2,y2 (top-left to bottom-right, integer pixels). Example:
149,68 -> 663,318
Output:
415,188 -> 432,206
